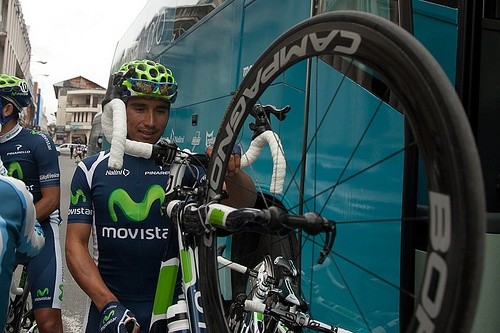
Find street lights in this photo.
[15,60,47,78]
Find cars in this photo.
[54,143,86,156]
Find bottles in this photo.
[166,299,190,333]
[165,200,179,217]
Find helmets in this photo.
[113,59,177,103]
[0,73,32,113]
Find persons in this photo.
[63,61,256,333]
[0,74,64,333]
[70,145,87,159]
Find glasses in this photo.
[125,77,178,96]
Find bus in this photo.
[104,0,500,332]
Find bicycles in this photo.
[99,9,486,333]
